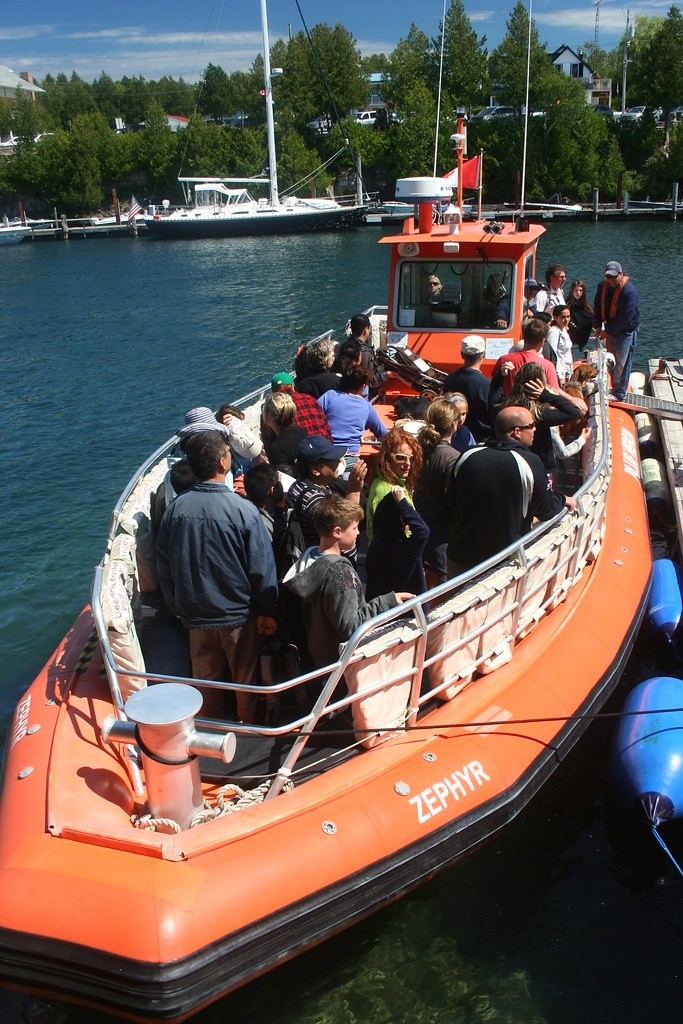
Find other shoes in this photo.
[608,393,621,401]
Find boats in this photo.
[0,110,661,1024]
[502,203,584,212]
[382,201,472,217]
[0,211,152,244]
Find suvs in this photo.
[588,105,613,119]
[470,105,521,122]
[348,111,379,124]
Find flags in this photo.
[127,196,144,227]
[443,154,481,189]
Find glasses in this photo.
[391,453,415,464]
[606,272,620,280]
[554,274,566,279]
[508,420,536,431]
[487,279,502,286]
[260,404,268,413]
[428,282,439,286]
[217,444,232,465]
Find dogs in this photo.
[561,364,600,438]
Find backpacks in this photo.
[275,485,328,569]
[253,641,328,733]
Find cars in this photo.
[116,124,145,135]
[522,106,549,118]
[0,132,53,146]
[615,106,683,121]
[202,116,266,127]
[307,114,331,132]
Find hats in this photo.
[461,336,485,355]
[272,371,298,394]
[176,406,229,439]
[604,261,622,276]
[525,278,540,292]
[297,434,348,467]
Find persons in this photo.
[592,262,641,400]
[152,263,592,730]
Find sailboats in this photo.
[144,1,381,237]
